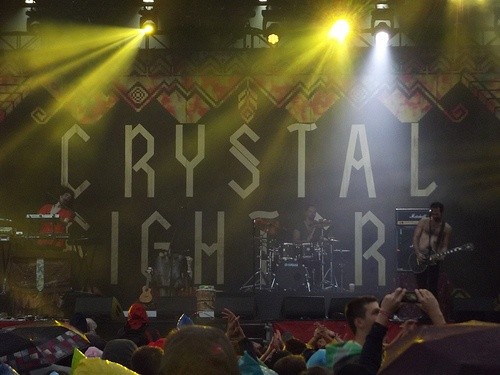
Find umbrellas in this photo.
[0,314,93,373]
[375,319,500,375]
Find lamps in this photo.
[25,6,43,34]
[371,2,394,42]
[261,1,284,45]
[138,2,160,35]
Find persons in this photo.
[0,284,500,375]
[294,206,329,243]
[33,184,77,257]
[410,198,454,294]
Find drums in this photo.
[297,242,315,259]
[277,241,296,261]
[274,260,312,293]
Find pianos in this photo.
[9,212,102,295]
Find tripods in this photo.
[323,243,337,289]
[241,245,270,293]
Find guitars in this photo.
[408,242,475,273]
[138,268,153,303]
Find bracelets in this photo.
[378,307,394,320]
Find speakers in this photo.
[74,296,124,319]
[216,297,260,319]
[279,295,376,319]
[396,226,431,292]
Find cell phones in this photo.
[401,292,417,303]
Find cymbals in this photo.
[309,221,331,226]
[261,222,287,235]
